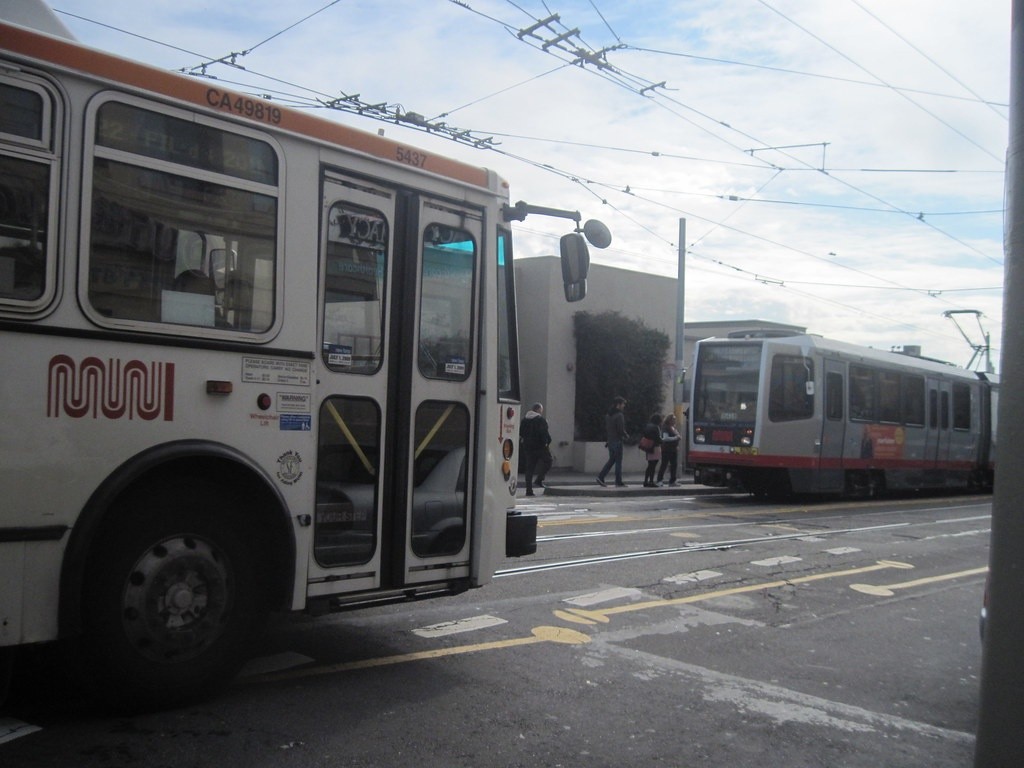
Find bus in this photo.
[0,0,611,725]
[678,308,1000,508]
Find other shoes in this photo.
[616,483,625,487]
[644,482,648,487]
[648,483,659,487]
[596,476,607,487]
[657,482,663,486]
[534,479,546,488]
[526,490,534,496]
[669,482,681,486]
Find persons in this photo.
[178,269,234,331]
[519,403,553,495]
[657,414,681,486]
[597,396,629,487]
[644,413,660,487]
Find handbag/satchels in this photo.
[639,436,656,453]
